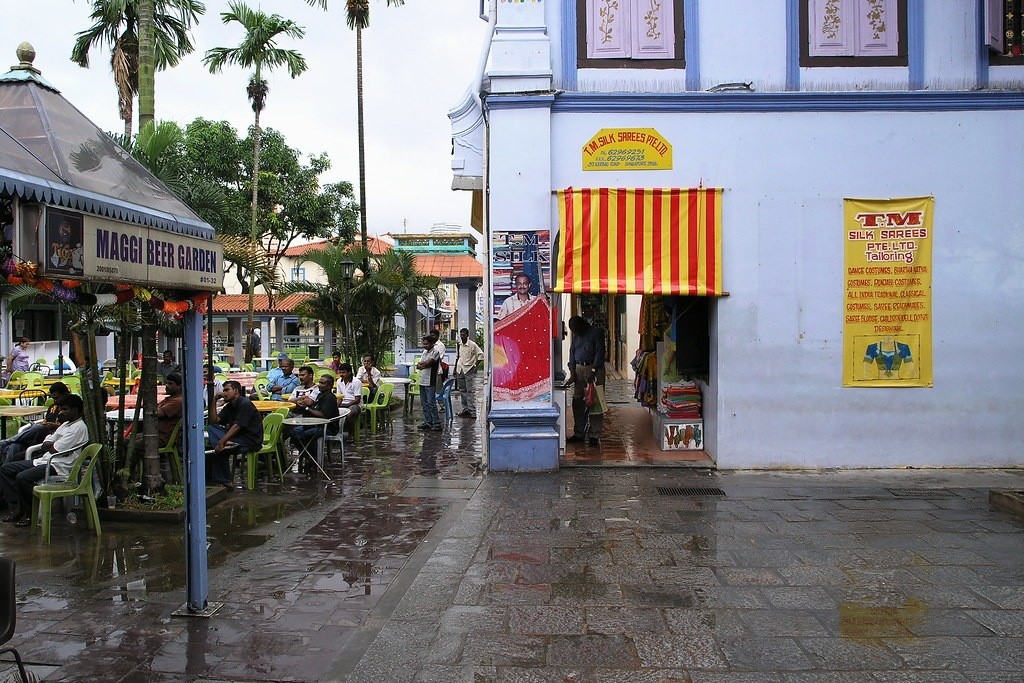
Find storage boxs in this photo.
[652,410,705,451]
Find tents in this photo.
[0,42,225,618]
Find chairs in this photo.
[0,347,455,545]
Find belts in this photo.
[577,361,593,365]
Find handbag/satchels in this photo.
[441,362,449,378]
[584,381,607,415]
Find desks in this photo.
[0,406,49,440]
[381,378,411,427]
[105,395,170,437]
[104,379,137,395]
[225,373,257,378]
[0,389,50,405]
[251,400,296,474]
[104,408,143,481]
[8,380,60,402]
[229,378,256,394]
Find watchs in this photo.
[591,368,597,372]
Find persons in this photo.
[111,349,263,486]
[661,301,676,383]
[415,328,445,431]
[248,351,381,469]
[564,316,606,446]
[3,337,30,388]
[215,326,261,365]
[498,272,550,321]
[0,382,108,527]
[454,328,483,418]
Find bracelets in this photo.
[305,407,308,411]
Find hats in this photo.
[278,353,287,359]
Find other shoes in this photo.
[1,510,22,521]
[455,412,470,417]
[216,482,234,490]
[431,427,443,431]
[589,437,599,446]
[218,443,241,456]
[16,513,31,527]
[567,434,585,440]
[347,433,354,442]
[417,423,433,429]
[471,413,477,417]
